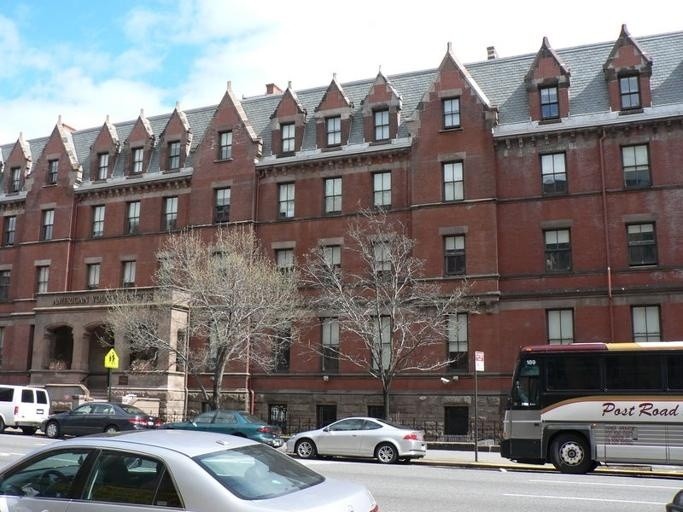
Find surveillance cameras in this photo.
[440,378,450,385]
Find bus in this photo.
[499,340,683,473]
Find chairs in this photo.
[244,465,267,480]
[98,455,128,475]
